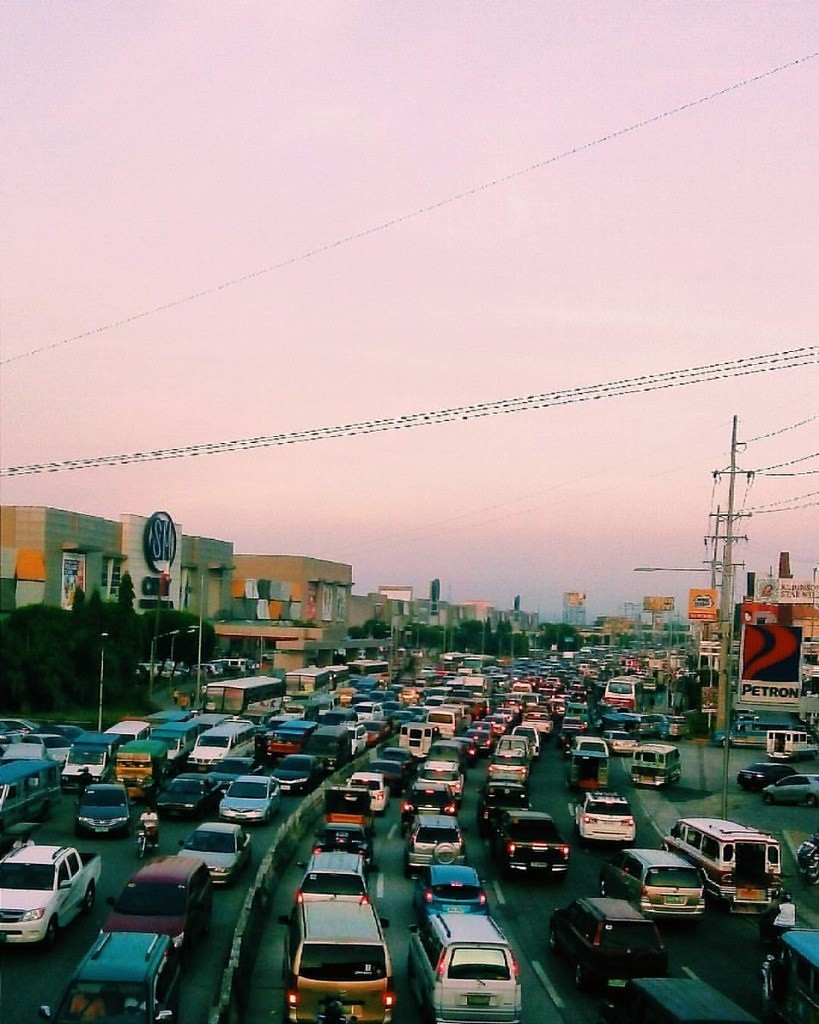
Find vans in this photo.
[277,899,396,1024]
[407,913,522,1023]
[632,743,682,788]
[105,720,152,752]
[0,759,61,834]
[602,967,762,1024]
[61,731,122,793]
[660,817,782,915]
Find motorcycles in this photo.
[137,821,155,858]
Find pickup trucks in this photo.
[496,810,571,877]
[1,844,100,953]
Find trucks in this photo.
[712,721,794,750]
[766,729,818,762]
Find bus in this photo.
[769,929,819,1024]
[115,739,168,803]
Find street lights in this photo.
[150,631,177,699]
[97,632,108,731]
[634,566,734,824]
[169,629,197,693]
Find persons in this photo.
[76,767,93,808]
[172,687,179,703]
[201,684,208,695]
[139,804,161,847]
[180,693,189,711]
[12,830,34,850]
[190,689,196,706]
[761,929,793,1017]
[759,888,797,928]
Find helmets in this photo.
[783,892,792,902]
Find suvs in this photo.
[403,814,468,877]
[477,782,532,836]
[399,781,461,825]
[412,864,492,925]
[549,897,671,990]
[311,823,377,871]
[599,848,707,928]
[293,852,372,904]
[37,931,182,1024]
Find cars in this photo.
[176,822,251,887]
[736,762,799,792]
[568,790,636,847]
[218,776,283,826]
[137,643,693,819]
[324,786,376,833]
[0,718,87,766]
[100,855,213,968]
[762,774,819,808]
[156,773,223,821]
[73,783,136,837]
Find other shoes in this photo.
[154,844,158,848]
